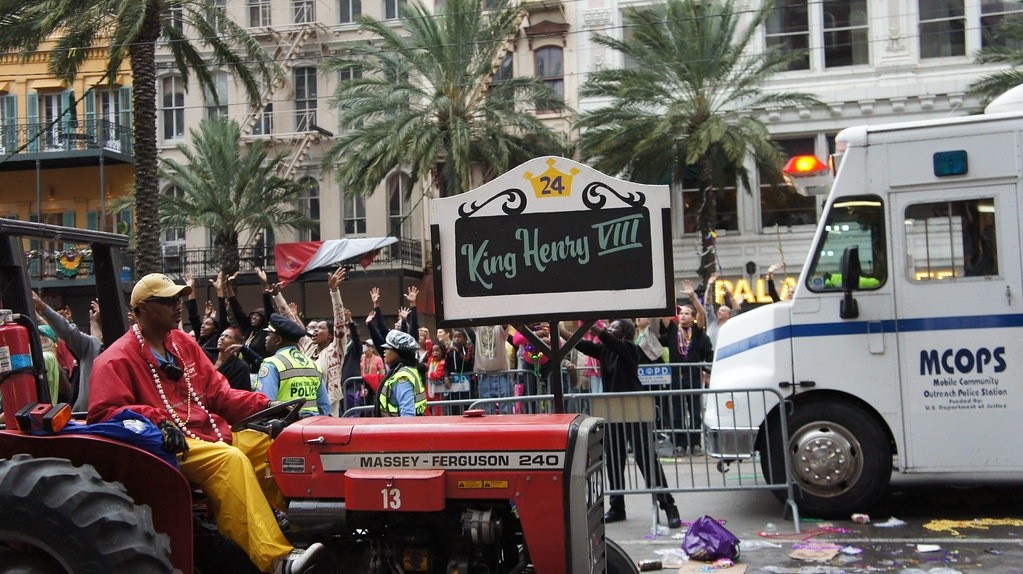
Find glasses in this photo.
[143,295,184,306]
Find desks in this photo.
[62,133,92,139]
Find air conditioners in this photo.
[162,245,179,258]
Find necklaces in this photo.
[132,323,223,441]
[487,326,494,360]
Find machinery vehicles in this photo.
[0,217,639,574]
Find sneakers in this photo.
[274,542,325,574]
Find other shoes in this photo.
[605,508,626,523]
[665,505,681,528]
[693,445,703,456]
[676,446,689,457]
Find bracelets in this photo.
[766,276,774,280]
[688,294,694,299]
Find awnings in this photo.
[87,75,123,87]
[31,79,66,90]
[0,82,9,93]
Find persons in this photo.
[340,309,362,416]
[559,319,681,529]
[635,273,740,457]
[360,339,385,417]
[766,265,795,302]
[181,267,346,416]
[375,329,426,416]
[472,324,510,416]
[366,285,477,416]
[505,320,606,414]
[32,291,103,414]
[86,274,324,574]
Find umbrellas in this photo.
[68,91,78,140]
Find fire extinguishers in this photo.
[0,309,47,430]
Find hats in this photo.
[38,324,57,342]
[129,272,193,311]
[249,307,266,315]
[380,329,421,353]
[362,338,375,348]
[262,313,305,343]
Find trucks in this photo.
[701,83,1022,519]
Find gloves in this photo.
[157,419,190,462]
[266,399,302,423]
